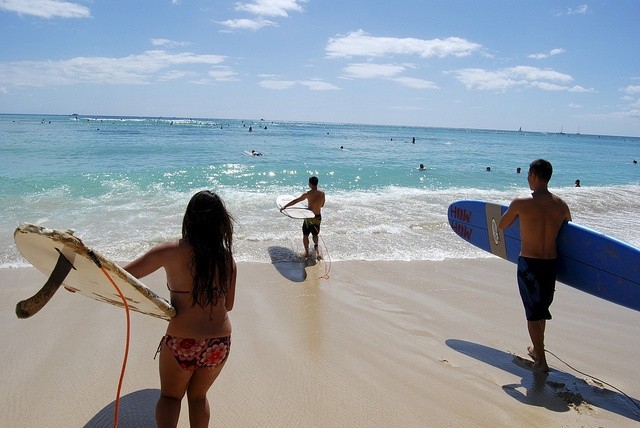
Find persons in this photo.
[419,164,426,170]
[486,166,490,171]
[412,136,416,143]
[252,150,262,156]
[499,159,572,372]
[574,179,580,187]
[340,145,343,149]
[517,168,521,173]
[63,190,237,427]
[279,177,325,256]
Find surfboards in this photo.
[276,194,315,219]
[15,222,178,321]
[447,200,640,312]
